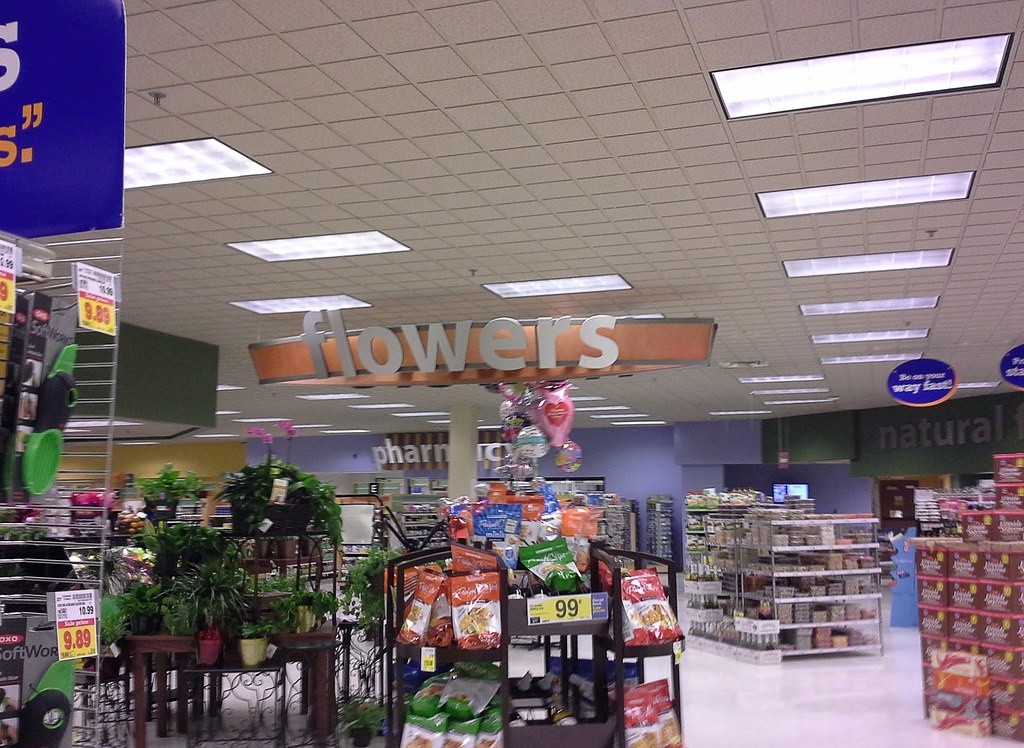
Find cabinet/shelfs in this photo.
[384,540,682,748]
[681,491,884,666]
[338,544,374,583]
[401,501,449,552]
[289,536,339,583]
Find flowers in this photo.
[212,418,296,509]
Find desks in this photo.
[86,555,341,748]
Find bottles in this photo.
[685,563,722,582]
[687,594,718,610]
[739,633,778,651]
[690,621,738,647]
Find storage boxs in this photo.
[914,452,1024,742]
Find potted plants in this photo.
[170,558,252,669]
[236,621,274,667]
[339,697,387,748]
[68,449,405,636]
[82,608,127,682]
